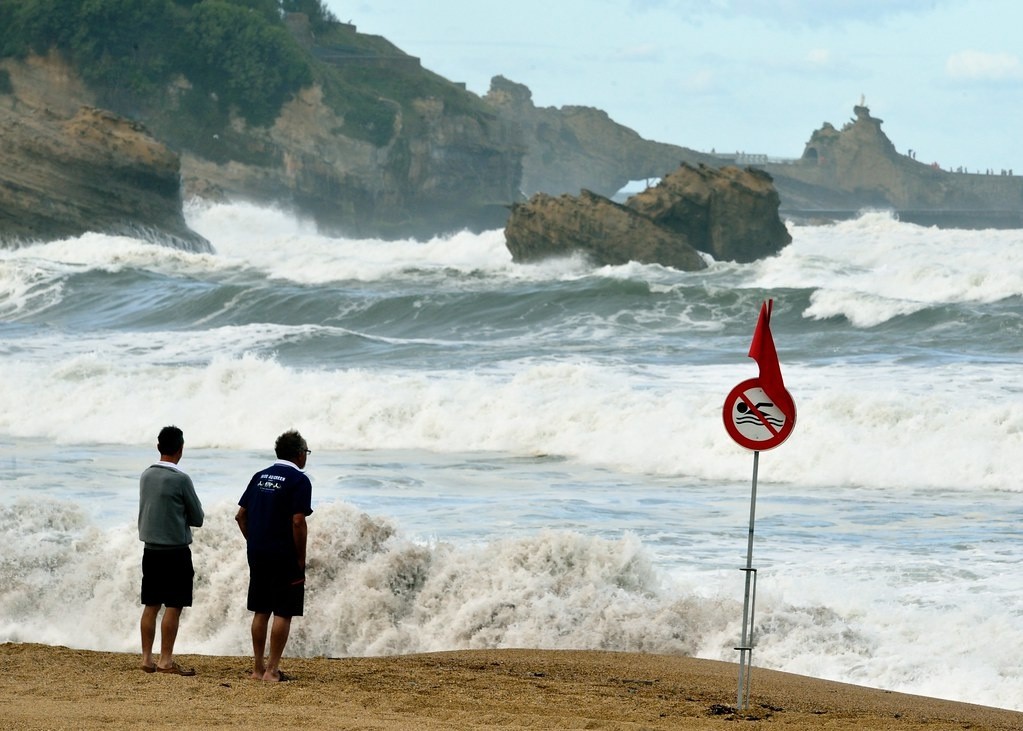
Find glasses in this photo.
[303,449,311,455]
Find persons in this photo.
[139,426,205,676]
[235,431,314,682]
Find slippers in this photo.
[277,670,292,681]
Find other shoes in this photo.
[157,660,195,676]
[141,662,158,673]
